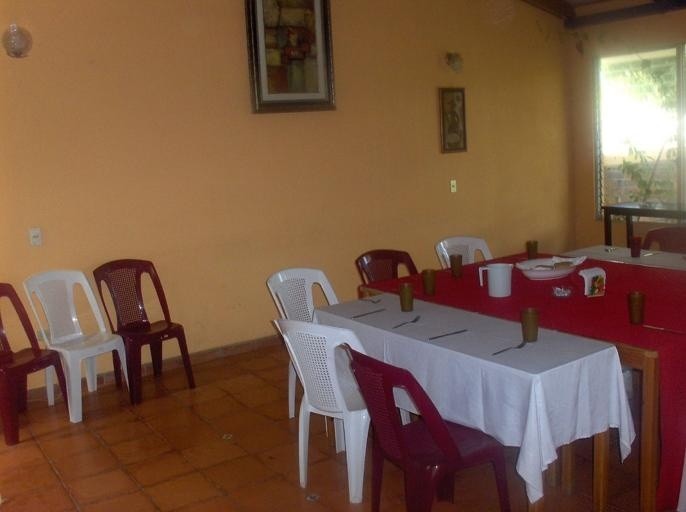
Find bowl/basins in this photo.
[515,255,587,281]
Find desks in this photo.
[600,200,685,250]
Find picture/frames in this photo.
[243,0,336,113]
[438,85,466,153]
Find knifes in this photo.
[643,324,686,336]
[642,251,661,257]
[426,329,469,340]
[350,308,388,319]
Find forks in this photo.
[490,342,526,356]
[390,314,420,329]
[357,297,381,304]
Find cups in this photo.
[450,254,462,278]
[627,291,645,324]
[521,307,539,343]
[630,235,641,258]
[525,240,538,259]
[397,283,414,312]
[550,279,573,297]
[603,240,616,257]
[420,269,437,295]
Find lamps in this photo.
[1,24,31,58]
[442,48,464,74]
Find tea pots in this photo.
[476,263,515,298]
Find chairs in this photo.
[0,283,68,449]
[20,270,132,427]
[94,259,193,404]
[643,226,685,251]
[267,267,340,419]
[273,318,410,504]
[340,342,511,512]
[355,249,418,283]
[436,236,493,269]
